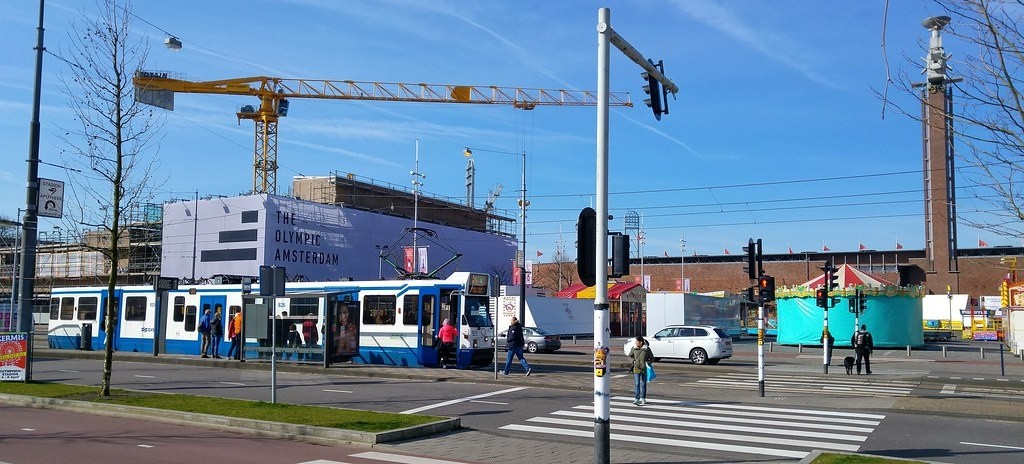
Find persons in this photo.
[498,316,531,377]
[199,309,247,361]
[259,311,319,362]
[821,324,873,375]
[375,308,460,369]
[628,336,655,405]
[332,303,357,356]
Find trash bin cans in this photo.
[81,323,92,351]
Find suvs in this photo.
[623,325,733,365]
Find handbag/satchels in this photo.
[198,320,204,332]
[646,362,657,382]
[629,366,633,373]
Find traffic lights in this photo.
[832,297,841,307]
[742,238,755,279]
[830,268,839,290]
[574,207,596,287]
[859,290,867,314]
[741,287,754,302]
[849,299,856,313]
[638,59,661,122]
[816,290,826,306]
[758,276,775,302]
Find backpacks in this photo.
[856,331,869,348]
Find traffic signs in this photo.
[37,178,65,219]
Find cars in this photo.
[494,326,561,353]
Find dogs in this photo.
[844,357,855,375]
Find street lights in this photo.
[462,146,527,327]
[15,0,182,383]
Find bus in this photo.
[47,228,495,370]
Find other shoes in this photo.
[443,365,447,369]
[633,399,640,404]
[642,399,646,404]
[213,356,215,358]
[215,355,221,358]
[857,371,860,374]
[525,367,532,376]
[202,354,210,358]
[226,357,230,360]
[867,371,872,375]
[499,370,508,377]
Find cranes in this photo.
[131,69,633,194]
